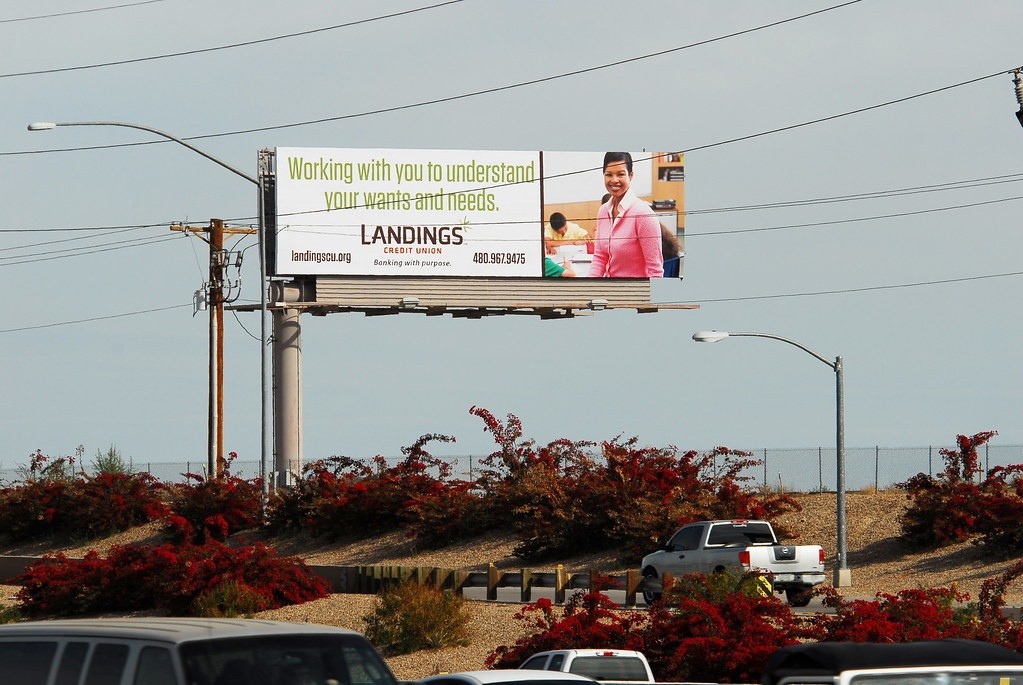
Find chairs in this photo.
[662,255,681,278]
[278,650,325,685]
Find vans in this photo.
[516,648,655,685]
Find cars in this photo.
[420,669,605,685]
[751,638,1023,685]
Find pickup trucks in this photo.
[641,518,826,608]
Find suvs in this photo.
[0,615,402,684]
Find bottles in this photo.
[585,241,595,254]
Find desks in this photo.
[652,208,678,238]
[545,246,592,277]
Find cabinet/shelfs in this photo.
[652,152,684,229]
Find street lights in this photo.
[27,122,272,525]
[691,329,851,588]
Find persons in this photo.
[602,194,680,278]
[588,152,664,278]
[544,212,592,277]
